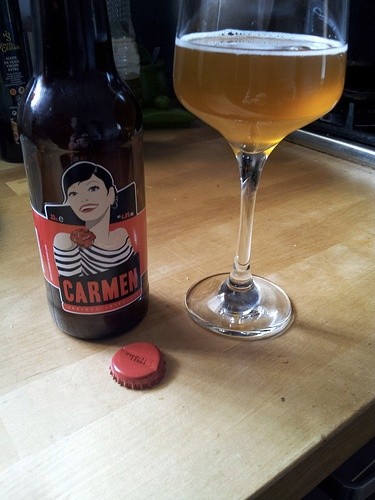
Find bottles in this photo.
[19,0,149,340]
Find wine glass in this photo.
[174,0,351,340]
